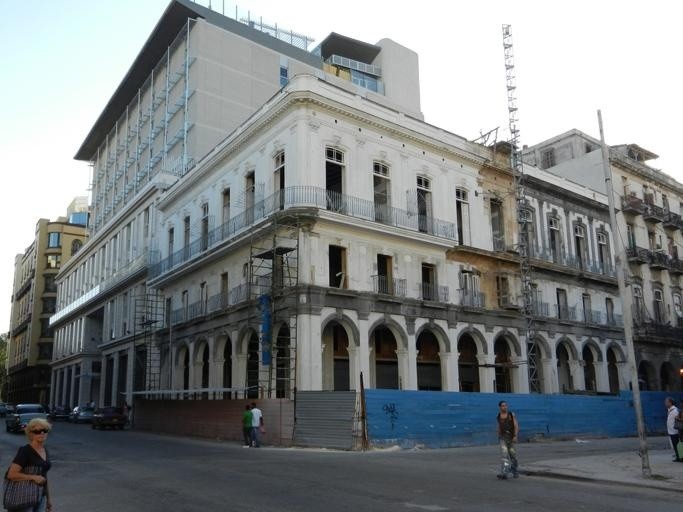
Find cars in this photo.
[0,402,127,433]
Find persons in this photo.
[4,417,52,512]
[241,405,254,449]
[250,401,264,448]
[496,400,521,479]
[88,400,95,410]
[123,401,130,425]
[665,395,683,463]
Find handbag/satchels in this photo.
[3,464,44,510]
[676,441,683,459]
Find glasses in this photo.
[29,427,48,434]
